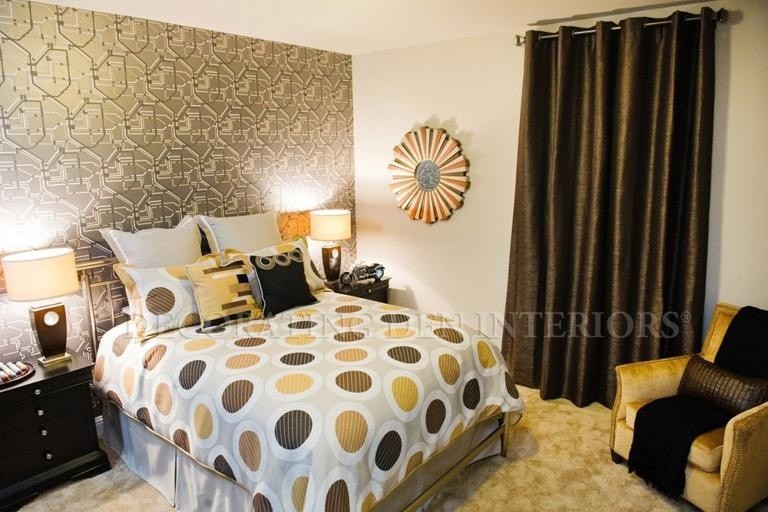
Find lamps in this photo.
[309,209,352,285]
[1,247,80,368]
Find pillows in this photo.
[223,234,334,294]
[183,256,262,331]
[250,247,319,319]
[194,211,283,255]
[112,254,223,342]
[99,214,202,265]
[247,272,265,307]
[678,353,768,413]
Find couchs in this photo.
[610,304,768,512]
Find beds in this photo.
[76,256,526,512]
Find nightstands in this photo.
[334,277,390,303]
[0,347,111,512]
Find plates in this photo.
[0,361,35,386]
